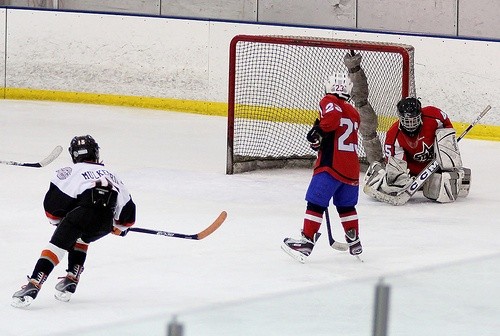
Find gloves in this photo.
[112,226,130,236]
[307,127,324,151]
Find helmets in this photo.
[68,135,100,164]
[325,73,354,100]
[396,98,421,137]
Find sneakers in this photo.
[8,282,41,308]
[53,274,79,302]
[345,228,364,263]
[281,231,322,263]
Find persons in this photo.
[283,72,365,257]
[7,135,138,302]
[384,98,455,191]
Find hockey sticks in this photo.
[129,211,229,241]
[363,104,492,207]
[312,139,349,252]
[0,146,63,167]
